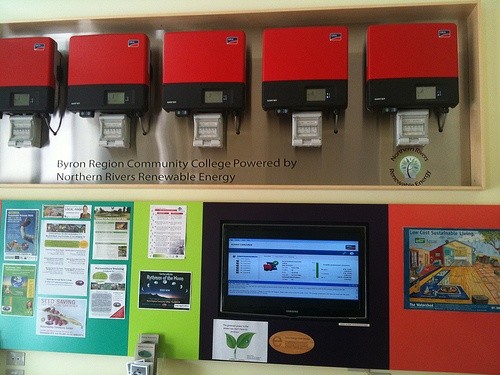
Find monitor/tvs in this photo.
[219,223,370,322]
